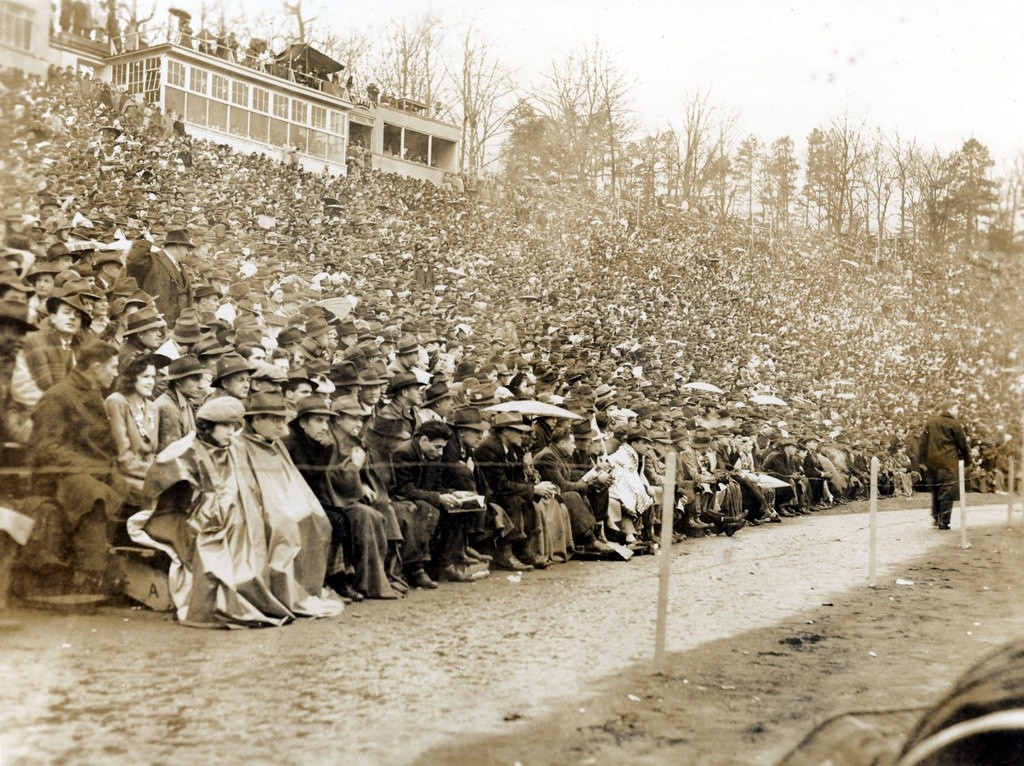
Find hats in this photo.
[0,64,814,443]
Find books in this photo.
[447,495,487,513]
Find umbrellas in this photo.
[683,383,722,393]
[315,297,352,320]
[749,395,788,406]
[478,400,585,447]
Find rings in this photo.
[450,504,454,508]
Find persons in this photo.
[917,399,977,529]
[0,0,1024,632]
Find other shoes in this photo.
[185,484,946,632]
